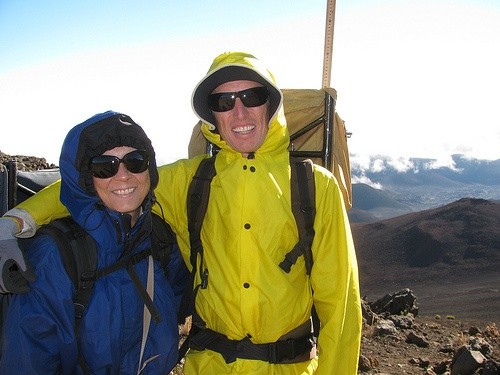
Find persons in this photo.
[0,110,194,375]
[0,51,362,375]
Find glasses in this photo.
[87,149,150,179]
[204,86,270,113]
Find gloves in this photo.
[1,218,39,292]
